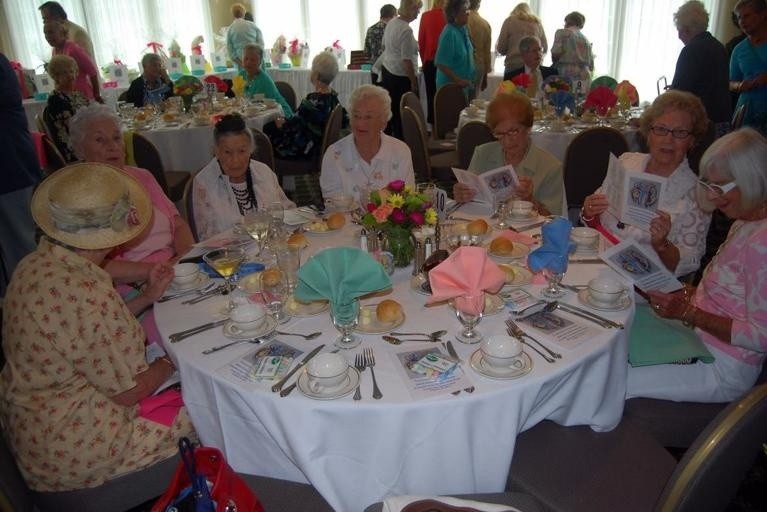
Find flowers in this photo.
[351,179,443,263]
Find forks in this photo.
[353,353,367,401]
[503,319,563,364]
[364,346,384,400]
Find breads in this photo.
[262,269,281,286]
[134,112,149,122]
[491,237,513,255]
[161,114,176,121]
[144,105,154,113]
[498,266,515,283]
[327,214,345,230]
[288,234,307,251]
[376,300,403,321]
[467,220,488,235]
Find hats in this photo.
[31,162,153,250]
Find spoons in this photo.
[379,335,443,345]
[390,329,448,338]
[275,330,323,341]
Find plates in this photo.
[295,364,361,400]
[319,201,361,212]
[451,223,494,236]
[166,270,211,293]
[502,212,538,221]
[278,295,329,318]
[350,302,406,335]
[444,293,506,317]
[469,348,534,380]
[572,248,601,257]
[492,263,534,285]
[221,313,280,340]
[487,240,530,259]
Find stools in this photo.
[234,468,333,511]
[364,493,545,512]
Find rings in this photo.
[653,304,662,311]
[590,206,591,208]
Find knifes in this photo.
[576,288,631,311]
[270,344,325,394]
[554,301,625,330]
[441,340,475,395]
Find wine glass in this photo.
[203,201,302,325]
[539,263,570,298]
[454,297,484,344]
[327,298,362,349]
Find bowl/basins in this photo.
[445,233,481,252]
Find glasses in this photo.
[650,127,691,139]
[493,129,518,139]
[698,179,736,195]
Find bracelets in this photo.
[156,355,175,372]
[581,207,594,221]
[681,303,691,322]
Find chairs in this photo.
[1,434,186,512]
[398,71,651,206]
[623,356,767,449]
[500,382,765,510]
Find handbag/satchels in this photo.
[151,436,262,512]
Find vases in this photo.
[377,231,420,267]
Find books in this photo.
[218,339,305,392]
[598,232,686,295]
[390,342,471,404]
[515,305,603,350]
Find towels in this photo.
[627,303,717,369]
[527,216,582,277]
[293,244,395,324]
[423,244,509,315]
[170,74,246,101]
[196,260,265,280]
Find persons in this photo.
[2,2,337,192]
[621,124,767,407]
[671,0,766,170]
[192,112,296,243]
[365,0,593,179]
[0,159,202,512]
[321,85,414,209]
[576,79,713,277]
[454,91,563,217]
[68,103,193,350]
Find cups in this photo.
[508,201,533,216]
[172,263,201,284]
[321,193,355,209]
[228,303,266,335]
[588,277,631,302]
[567,227,600,248]
[464,95,632,134]
[479,334,526,370]
[115,92,278,130]
[306,352,350,393]
